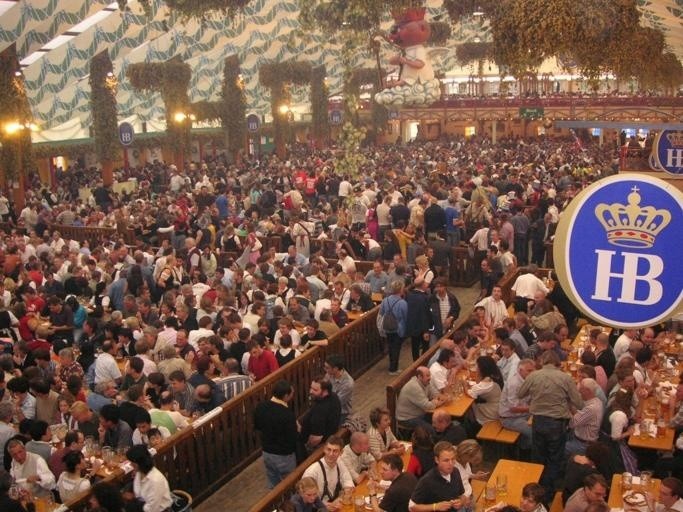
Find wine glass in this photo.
[584,324,592,344]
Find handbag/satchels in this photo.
[156,268,174,289]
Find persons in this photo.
[2,131,683,511]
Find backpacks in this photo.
[383,297,403,330]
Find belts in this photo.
[573,434,592,445]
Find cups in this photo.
[350,303,358,316]
[483,474,507,506]
[9,483,56,512]
[84,434,118,471]
[619,471,652,494]
[634,332,682,440]
[447,348,494,400]
[340,486,366,512]
[566,343,583,377]
[327,282,334,290]
